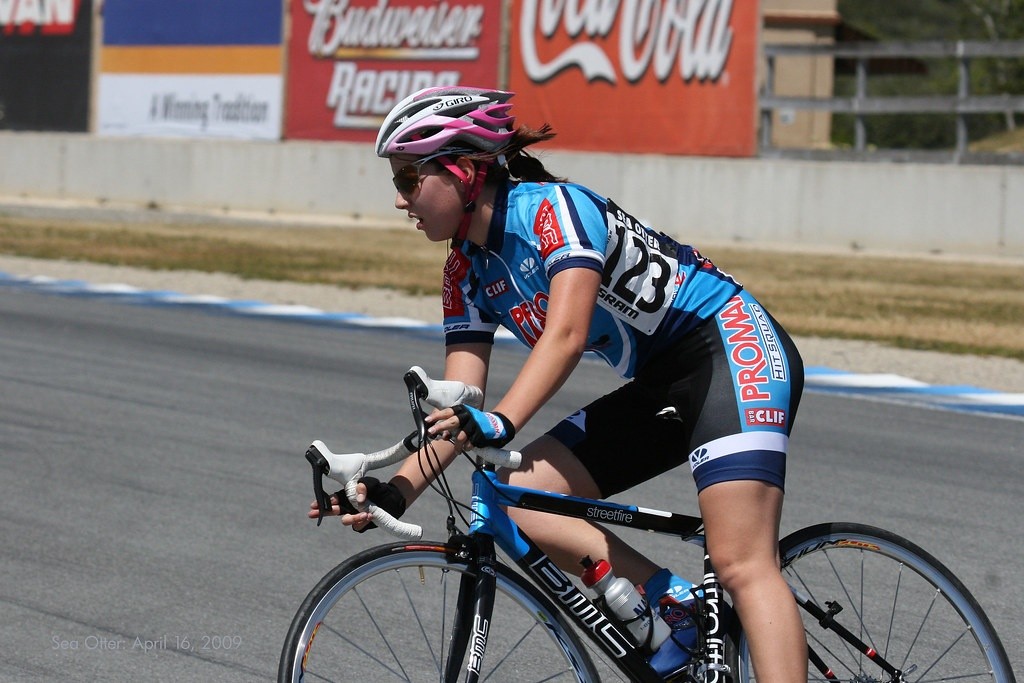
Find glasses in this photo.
[392,150,472,194]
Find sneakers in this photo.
[642,567,712,679]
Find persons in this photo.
[310,86,809,683]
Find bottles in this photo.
[579,555,672,652]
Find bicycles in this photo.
[276,363,1016,683]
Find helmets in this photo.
[374,86,521,158]
